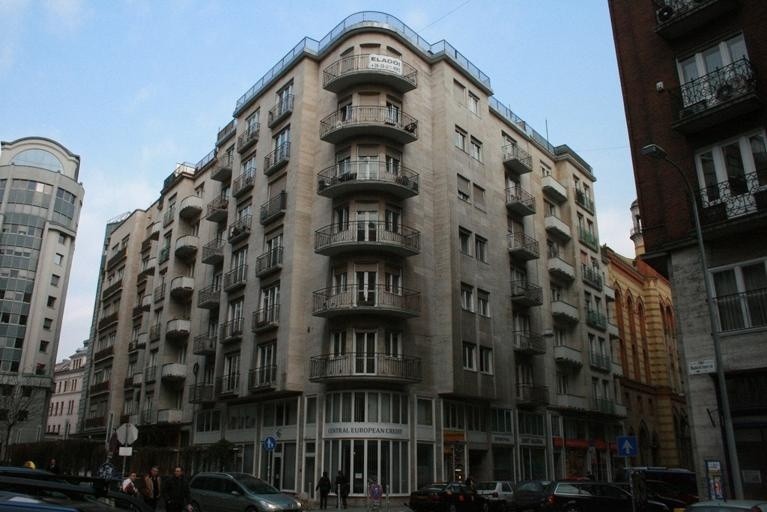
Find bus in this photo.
[615,466,698,512]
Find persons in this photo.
[47,459,60,474]
[163,466,193,512]
[315,471,332,510]
[334,471,349,509]
[120,471,138,496]
[142,464,162,512]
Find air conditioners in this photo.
[654,3,678,25]
[678,73,748,122]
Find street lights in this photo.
[641,143,745,499]
